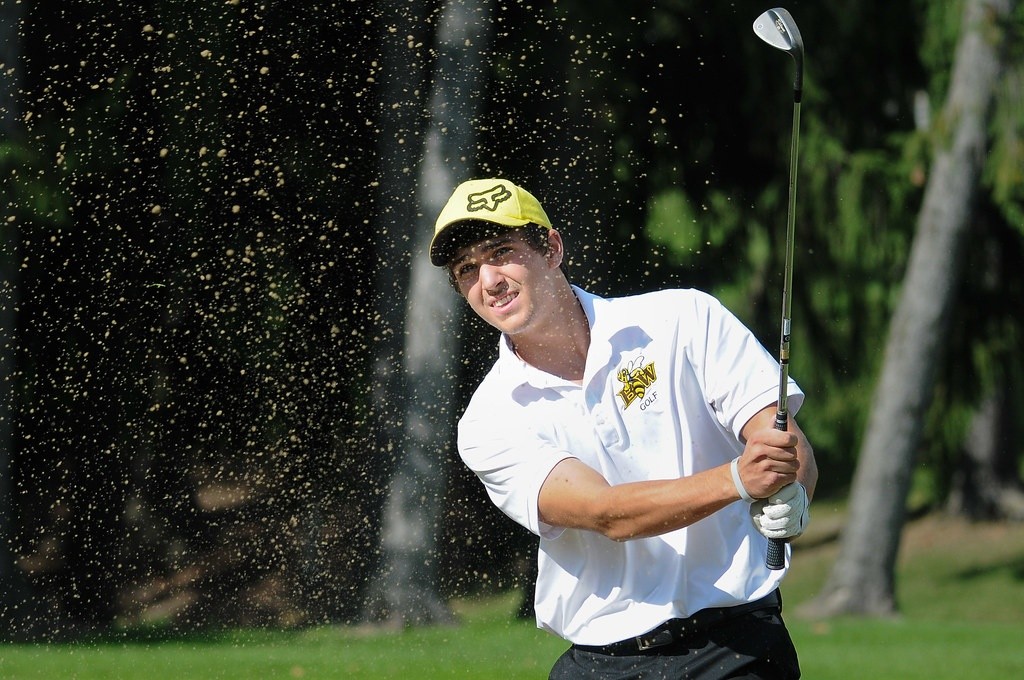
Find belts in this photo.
[594,589,782,654]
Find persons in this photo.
[429,179,818,680]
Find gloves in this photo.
[749,479,812,540]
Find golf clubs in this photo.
[751,7,805,572]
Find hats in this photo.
[430,178,554,268]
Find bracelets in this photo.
[730,456,758,503]
